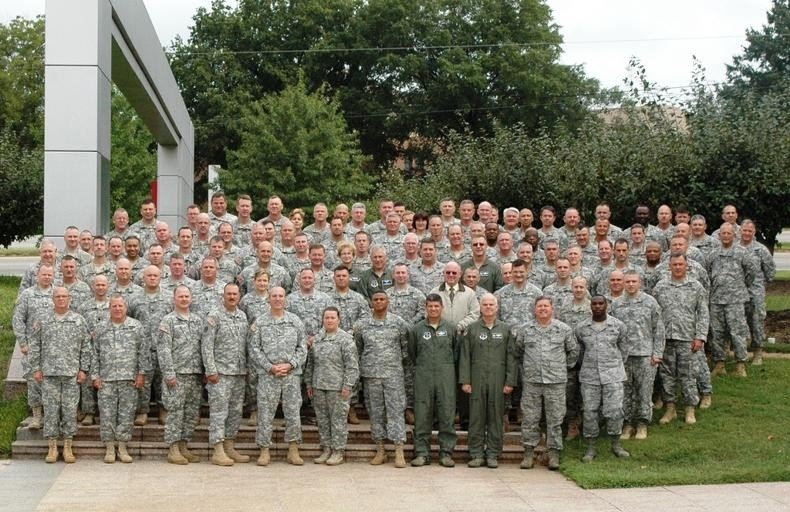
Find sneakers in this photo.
[411,456,431,466]
[135,413,148,425]
[81,413,94,425]
[620,424,634,439]
[212,439,250,465]
[247,411,257,426]
[565,425,579,440]
[736,361,747,377]
[349,408,359,423]
[711,362,726,375]
[158,407,167,425]
[636,421,647,439]
[468,459,484,467]
[487,457,497,467]
[439,454,455,466]
[314,447,343,464]
[653,395,711,424]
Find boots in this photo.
[370,445,388,464]
[257,447,270,465]
[286,441,303,465]
[395,444,406,468]
[752,348,763,365]
[548,449,560,469]
[29,406,42,429]
[63,439,76,462]
[520,447,533,468]
[168,439,200,464]
[104,441,116,463]
[582,437,598,461]
[117,441,133,462]
[612,435,630,457]
[45,437,58,462]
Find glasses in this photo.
[474,242,484,245]
[446,271,457,275]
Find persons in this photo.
[12,192,777,470]
